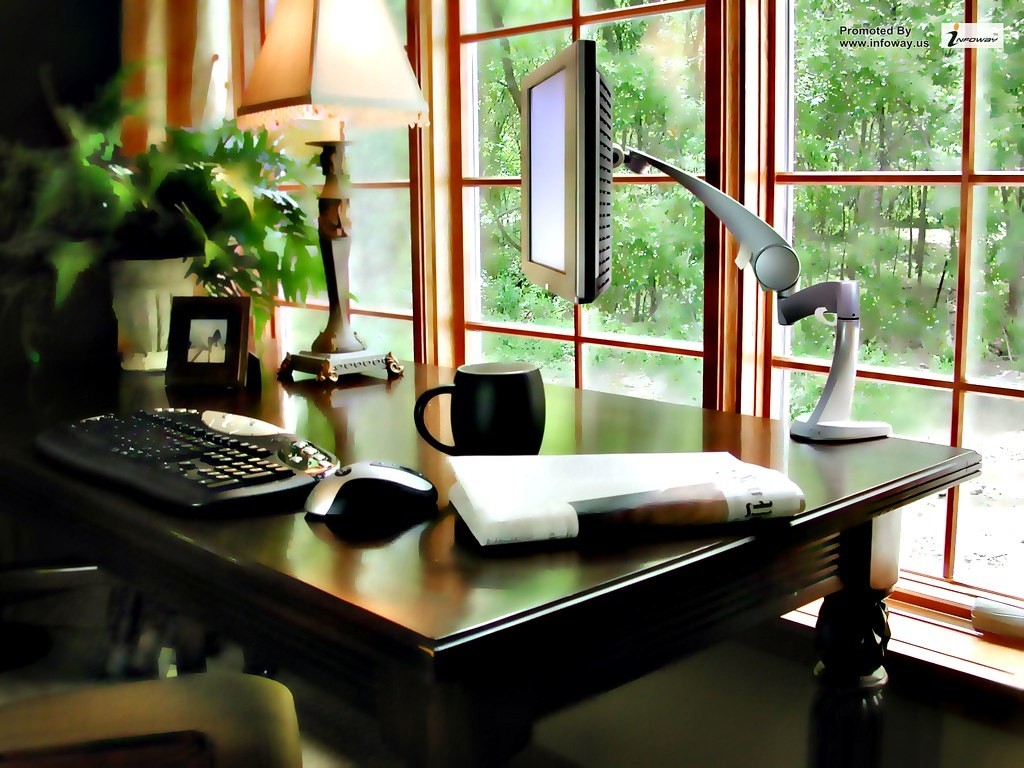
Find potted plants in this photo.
[0,55,327,377]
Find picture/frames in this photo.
[161,295,251,386]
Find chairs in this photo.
[1,561,410,768]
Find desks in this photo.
[1,338,979,768]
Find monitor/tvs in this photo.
[521,39,614,302]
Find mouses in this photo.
[305,460,440,519]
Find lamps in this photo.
[234,0,431,385]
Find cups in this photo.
[414,362,545,455]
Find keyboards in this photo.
[35,408,341,519]
